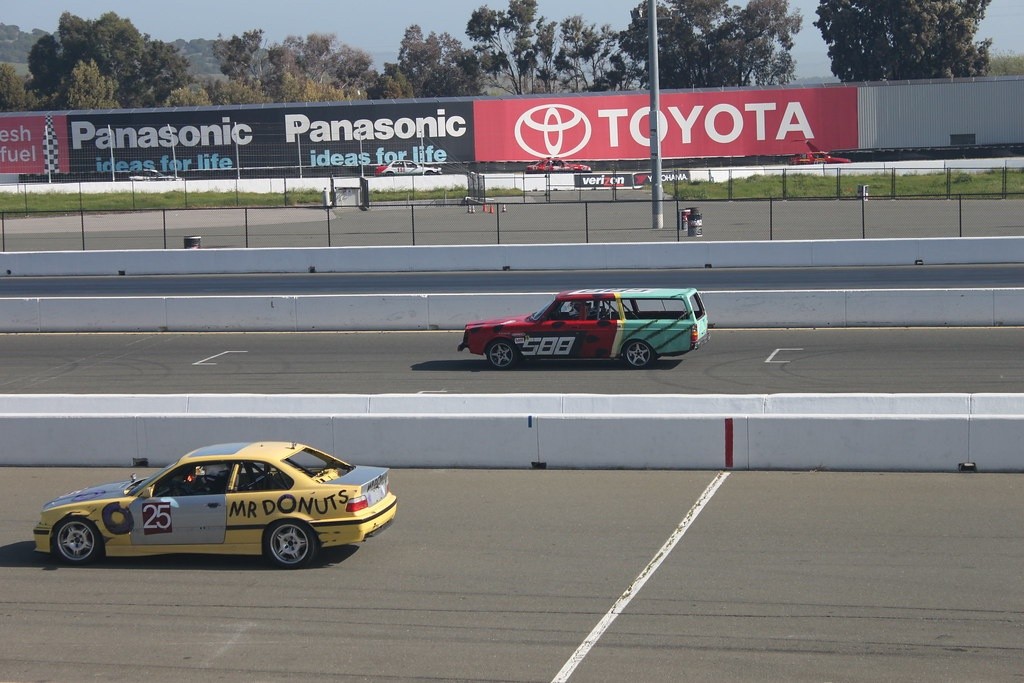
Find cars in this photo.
[129,169,185,181]
[526,157,592,174]
[457,287,711,370]
[791,152,850,165]
[33,441,397,568]
[375,160,442,176]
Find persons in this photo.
[200,464,242,492]
[568,300,589,319]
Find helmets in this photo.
[568,301,584,316]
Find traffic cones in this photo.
[472,205,475,213]
[468,205,471,213]
[502,204,506,212]
